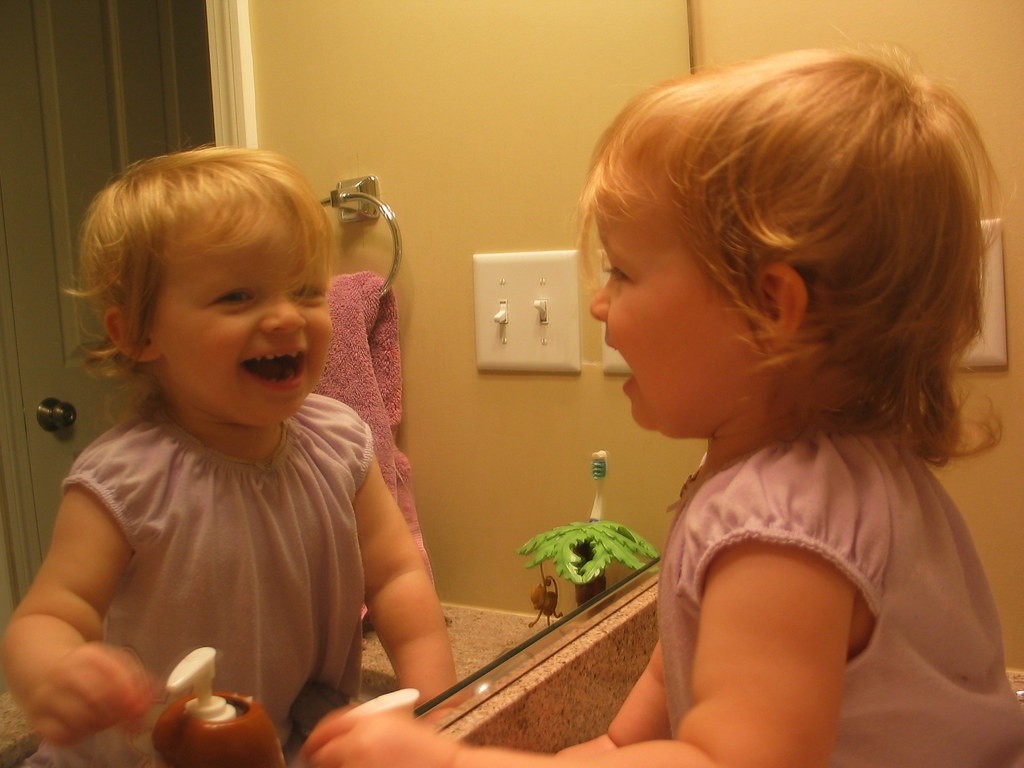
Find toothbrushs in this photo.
[587,449,609,525]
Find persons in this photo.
[6,145,457,768]
[293,40,1024,768]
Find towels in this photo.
[305,272,424,623]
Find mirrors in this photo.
[0,0,708,768]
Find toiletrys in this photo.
[149,645,285,768]
